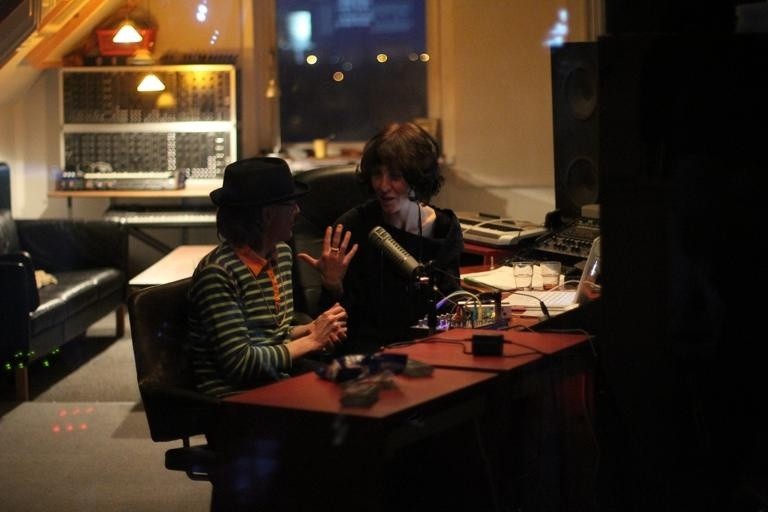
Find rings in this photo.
[330,247,340,253]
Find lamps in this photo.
[112,1,142,44]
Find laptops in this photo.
[501,237,602,310]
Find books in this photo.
[458,264,579,320]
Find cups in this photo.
[539,261,562,290]
[511,260,533,292]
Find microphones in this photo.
[368,226,425,281]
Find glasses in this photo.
[269,202,296,216]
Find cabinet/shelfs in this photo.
[58,63,238,188]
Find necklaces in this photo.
[229,242,287,327]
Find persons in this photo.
[164,153,349,512]
[294,118,465,362]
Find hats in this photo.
[209,157,310,208]
